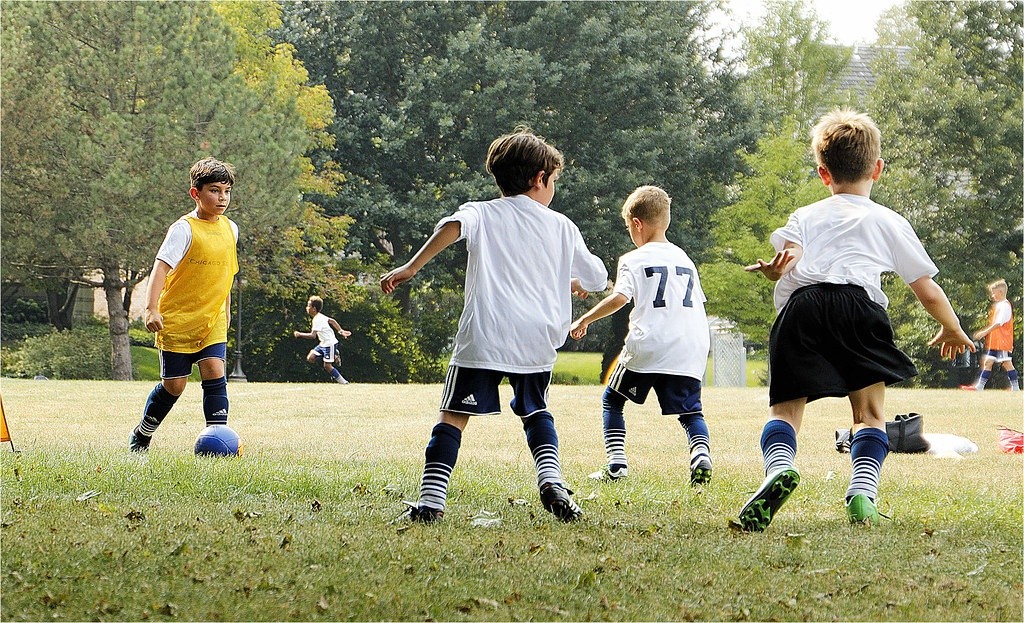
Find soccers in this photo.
[194,424,244,459]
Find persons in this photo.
[293,294,351,385]
[961,279,1020,393]
[127,156,239,459]
[567,184,712,489]
[380,126,608,534]
[737,106,976,537]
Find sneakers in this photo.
[739,466,800,533]
[392,501,444,525]
[130,425,149,457]
[334,349,341,367]
[845,494,892,528]
[540,481,583,523]
[603,463,628,484]
[961,384,977,391]
[690,455,712,489]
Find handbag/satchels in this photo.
[836,430,851,451]
[886,413,931,452]
